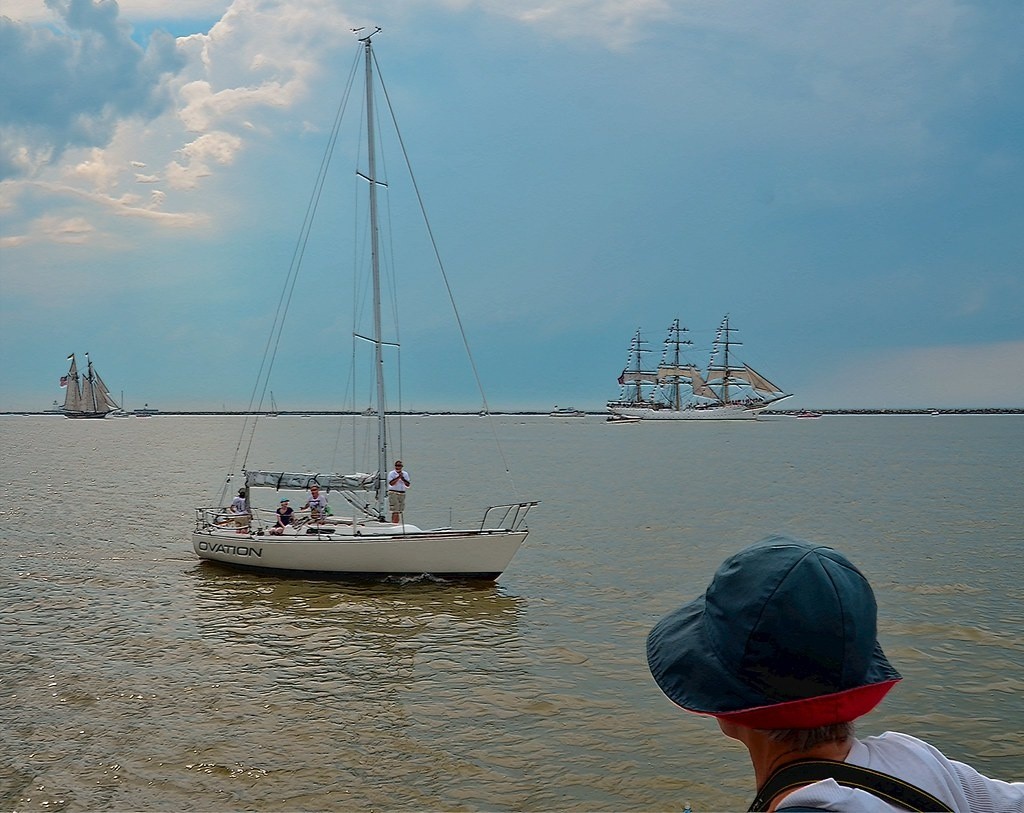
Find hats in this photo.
[238,488,245,493]
[280,497,290,503]
[647,536,904,728]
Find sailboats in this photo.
[189,25,543,585]
[57,352,160,420]
[604,311,795,421]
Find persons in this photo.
[230,488,247,533]
[300,486,328,524]
[647,534,1024,813]
[388,461,410,523]
[269,497,295,536]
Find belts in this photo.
[390,490,405,493]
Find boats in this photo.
[797,410,823,418]
[549,409,587,418]
[605,413,643,424]
